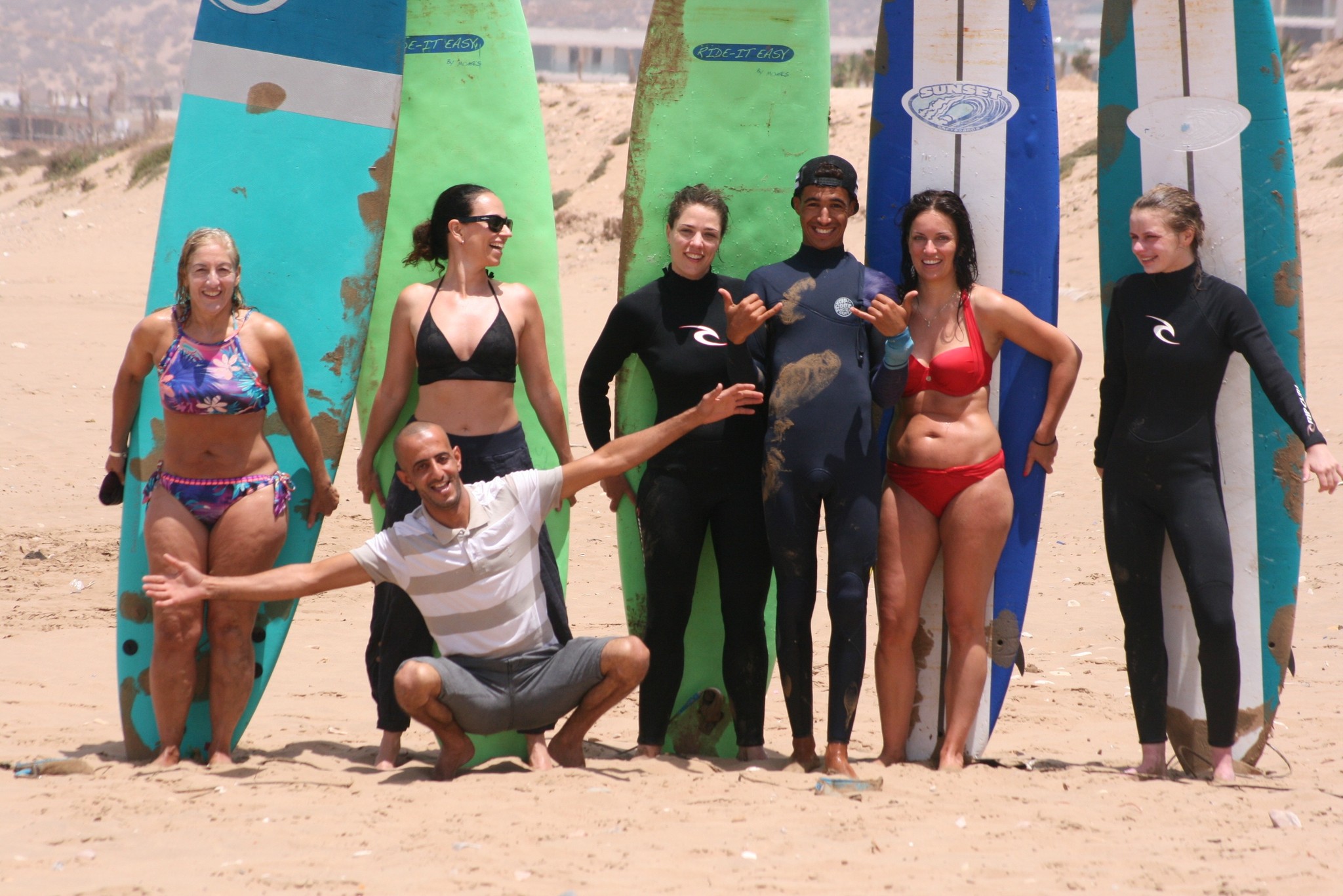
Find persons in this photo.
[356,184,578,775]
[1093,186,1341,786]
[105,227,339,776]
[141,383,765,781]
[579,184,774,761]
[874,187,1082,772]
[719,156,918,778]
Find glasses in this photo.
[460,215,513,232]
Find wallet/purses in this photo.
[99,471,123,506]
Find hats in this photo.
[791,155,860,216]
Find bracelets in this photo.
[108,445,127,458]
[1032,436,1056,446]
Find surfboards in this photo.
[864,1,1061,767]
[114,0,570,765]
[1094,2,1309,779]
[612,0,831,766]
